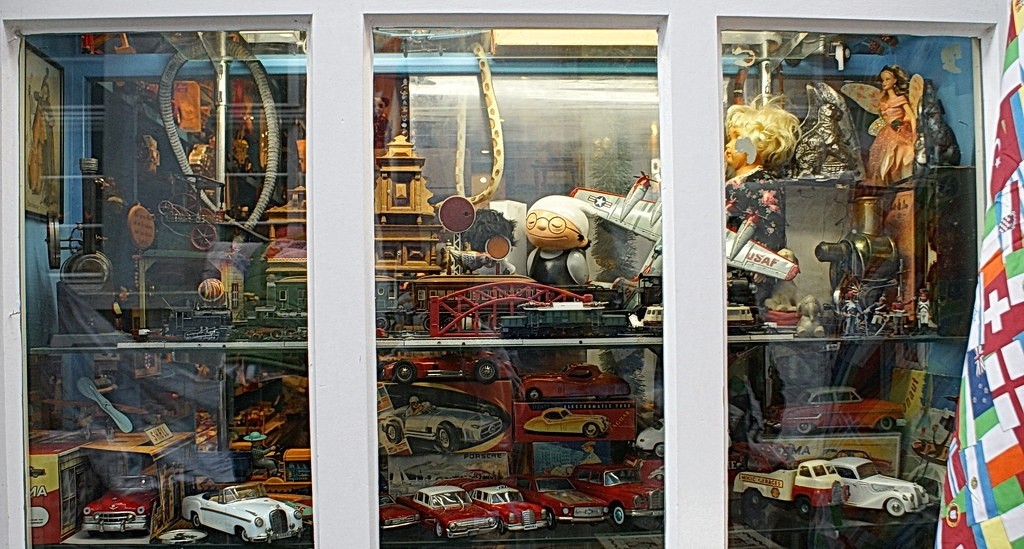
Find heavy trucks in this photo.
[732,459,846,520]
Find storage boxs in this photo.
[61,432,196,545]
[885,165,977,336]
[761,430,902,478]
[388,450,509,503]
[512,401,637,441]
[377,381,516,453]
[519,441,612,480]
[29,429,106,545]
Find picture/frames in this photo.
[24,40,64,225]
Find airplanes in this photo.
[41,375,149,440]
[570,171,800,310]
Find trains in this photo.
[161,273,540,341]
[495,301,756,339]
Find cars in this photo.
[379,492,419,531]
[763,385,906,433]
[181,482,303,543]
[501,472,609,529]
[571,462,664,525]
[80,474,158,535]
[518,361,632,401]
[635,419,733,456]
[828,457,933,518]
[466,484,548,534]
[382,350,517,383]
[396,485,499,539]
